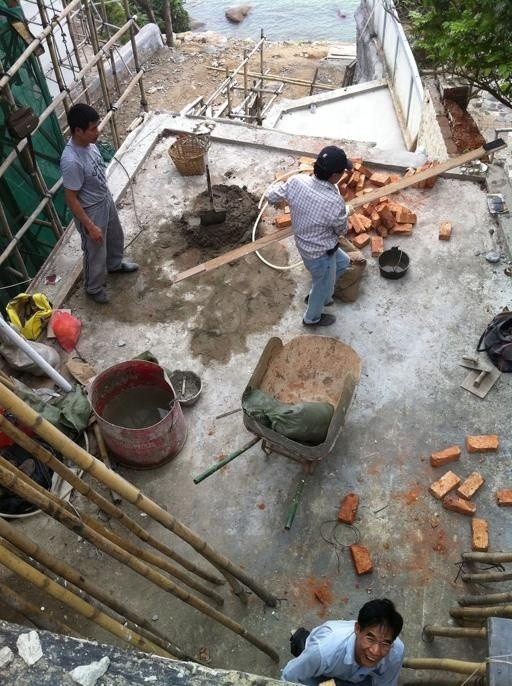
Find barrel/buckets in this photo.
[89,359,189,470]
[378,246,410,279]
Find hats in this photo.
[316,145,353,173]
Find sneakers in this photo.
[89,291,109,303]
[109,262,139,273]
[303,294,334,307]
[303,313,336,327]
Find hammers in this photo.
[88,415,122,504]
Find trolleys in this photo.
[194,334,363,530]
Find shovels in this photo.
[200,165,227,227]
[459,355,502,398]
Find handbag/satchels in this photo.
[4,292,53,342]
[477,310,511,374]
[335,255,368,304]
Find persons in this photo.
[59,102,141,303]
[264,144,355,327]
[281,597,406,685]
